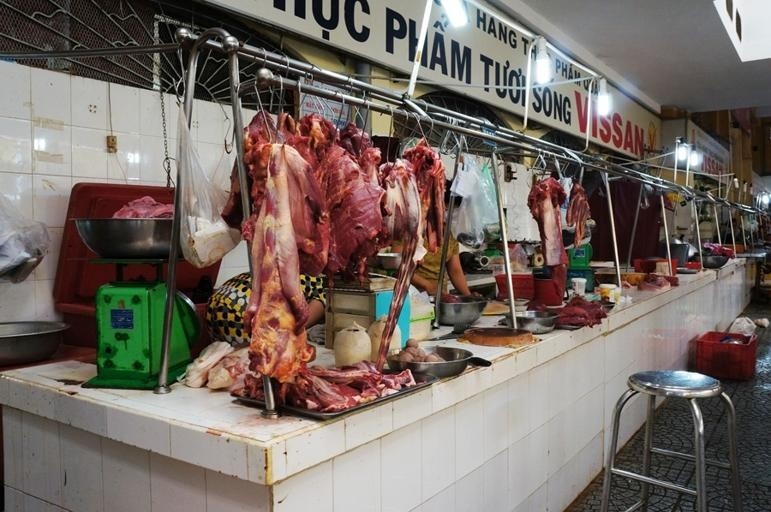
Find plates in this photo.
[675,267,698,274]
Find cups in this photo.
[600,284,619,306]
[570,278,587,297]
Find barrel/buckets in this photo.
[661,244,690,266]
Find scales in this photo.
[67,212,208,391]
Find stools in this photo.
[600,370,743,512]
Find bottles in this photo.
[333,327,372,368]
[368,319,402,364]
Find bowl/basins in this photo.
[431,294,491,326]
[694,255,731,267]
[387,345,475,381]
[0,320,71,366]
[502,311,560,334]
[503,297,531,307]
[366,251,402,269]
[685,262,702,271]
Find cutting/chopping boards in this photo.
[593,272,648,287]
[462,326,535,347]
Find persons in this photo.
[404,178,485,303]
